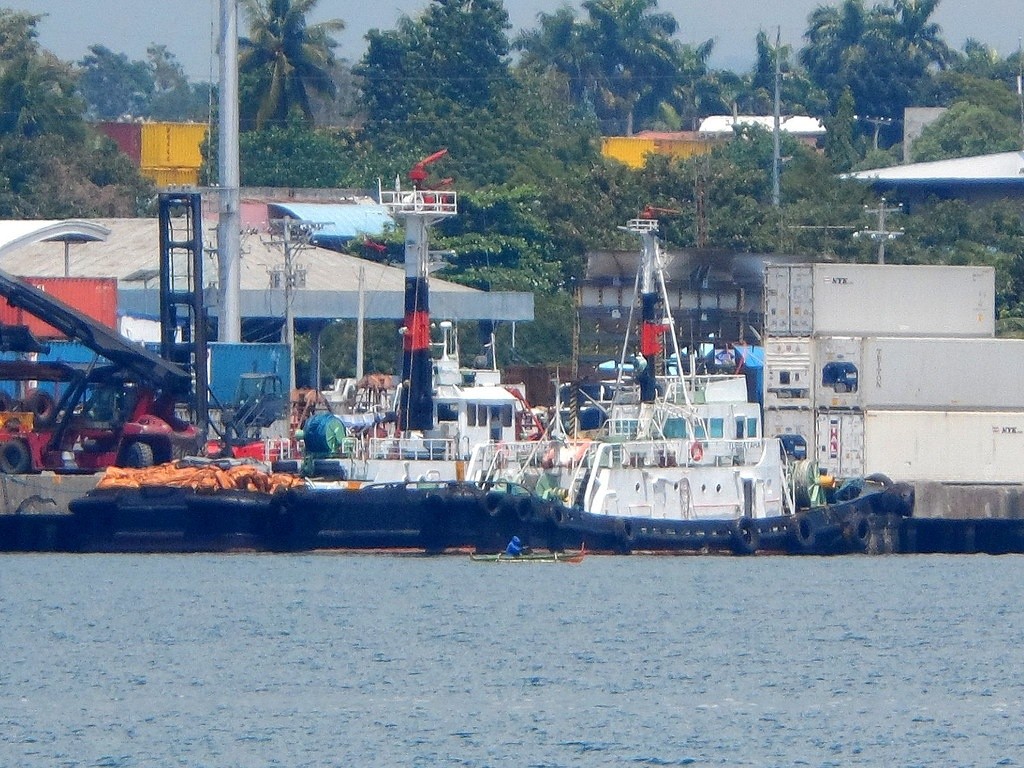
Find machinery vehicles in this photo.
[0,270,204,474]
[157,191,301,462]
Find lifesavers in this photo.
[731,516,760,555]
[851,512,871,545]
[546,503,567,529]
[484,491,504,518]
[691,442,705,462]
[789,513,817,548]
[614,515,637,546]
[901,486,915,517]
[514,497,534,524]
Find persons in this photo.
[541,436,566,474]
[491,416,502,442]
[506,536,526,557]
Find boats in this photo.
[67,147,592,555]
[468,542,585,562]
[265,208,917,555]
